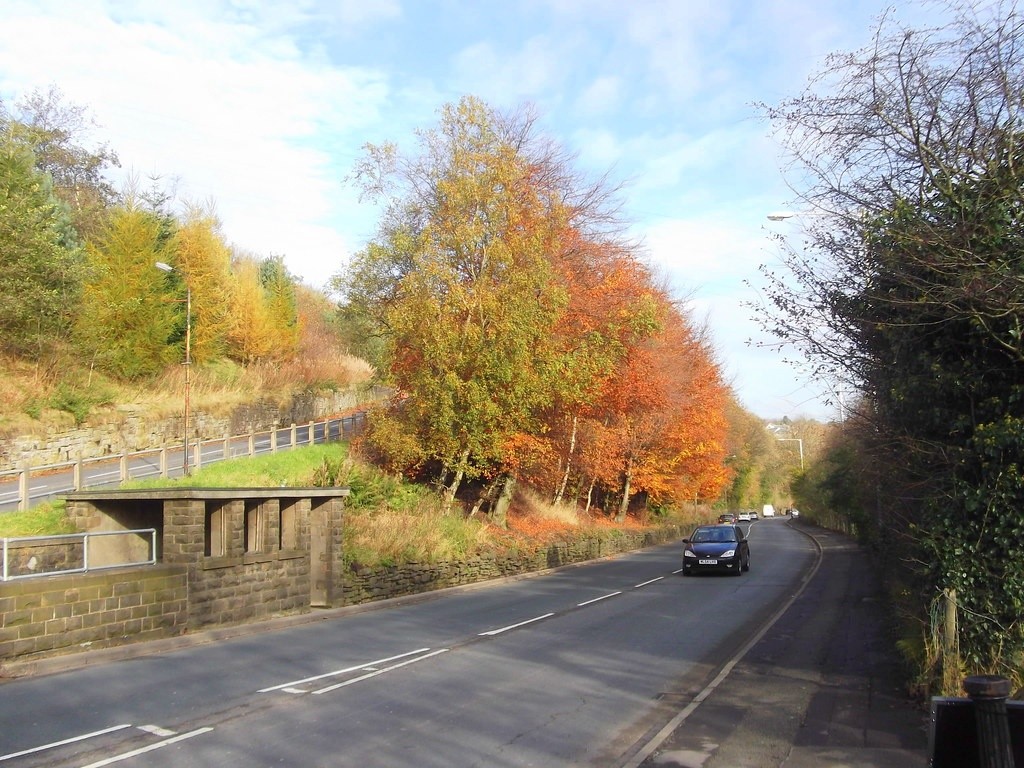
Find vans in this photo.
[763,505,774,517]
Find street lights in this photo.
[155,262,193,477]
[779,437,804,472]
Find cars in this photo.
[681,524,750,576]
[738,512,751,522]
[717,513,737,525]
[748,511,758,520]
